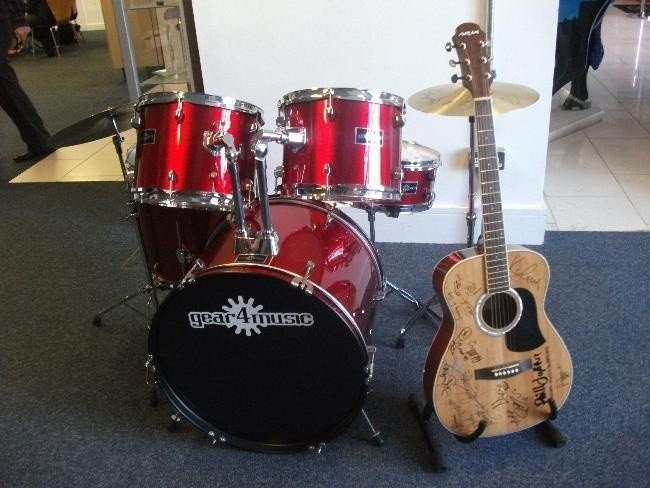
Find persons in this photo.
[1,1,57,164]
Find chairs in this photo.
[30,1,86,62]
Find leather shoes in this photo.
[15,149,53,162]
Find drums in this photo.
[145,194,388,453]
[123,142,263,288]
[275,86,405,203]
[349,140,442,218]
[130,90,265,213]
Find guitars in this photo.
[423,21,574,439]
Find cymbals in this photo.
[45,100,140,150]
[407,81,540,117]
